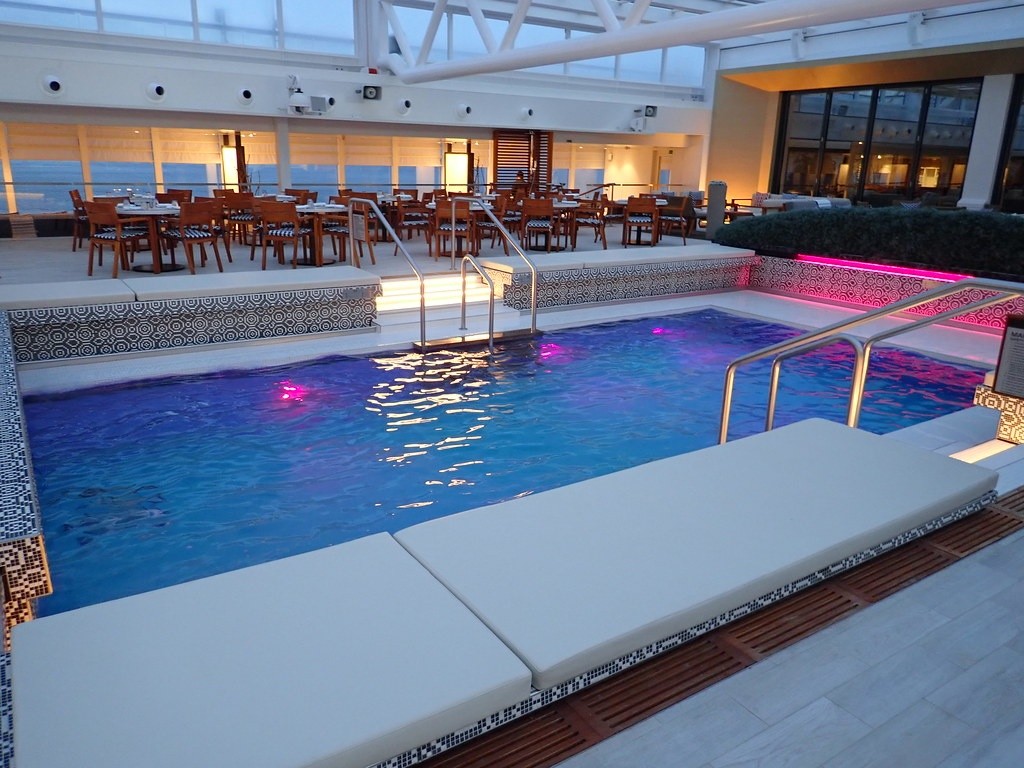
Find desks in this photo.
[617,200,667,245]
[762,200,790,215]
[424,203,494,257]
[116,207,184,272]
[371,197,413,242]
[290,205,349,265]
[517,202,579,252]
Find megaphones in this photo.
[362,85,382,101]
[641,105,658,118]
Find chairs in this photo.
[67,186,852,279]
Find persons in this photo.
[511,170,528,197]
